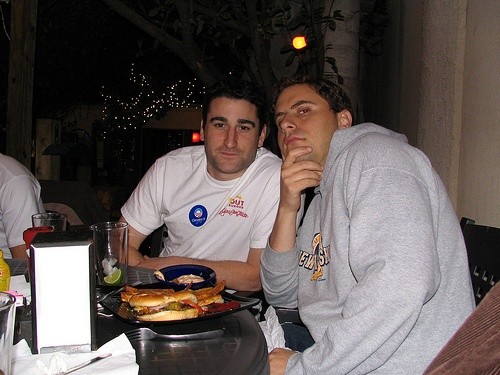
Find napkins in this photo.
[9,333,139,375]
[8,274,31,307]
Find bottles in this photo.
[0,249,11,292]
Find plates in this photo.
[98,281,261,327]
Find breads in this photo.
[121,280,224,306]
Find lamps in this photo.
[42,129,96,158]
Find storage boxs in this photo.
[29,232,97,355]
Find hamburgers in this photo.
[129,292,205,321]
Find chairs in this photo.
[36,180,111,230]
[459,218,500,307]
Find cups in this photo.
[32,212,68,231]
[89,221,130,287]
[0,291,17,375]
[22,225,55,274]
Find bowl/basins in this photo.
[154,264,216,290]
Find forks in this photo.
[125,327,225,342]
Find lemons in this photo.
[104,269,123,284]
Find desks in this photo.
[4,258,270,375]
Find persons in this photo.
[0,153,51,260]
[108,79,306,291]
[257,74,477,375]
